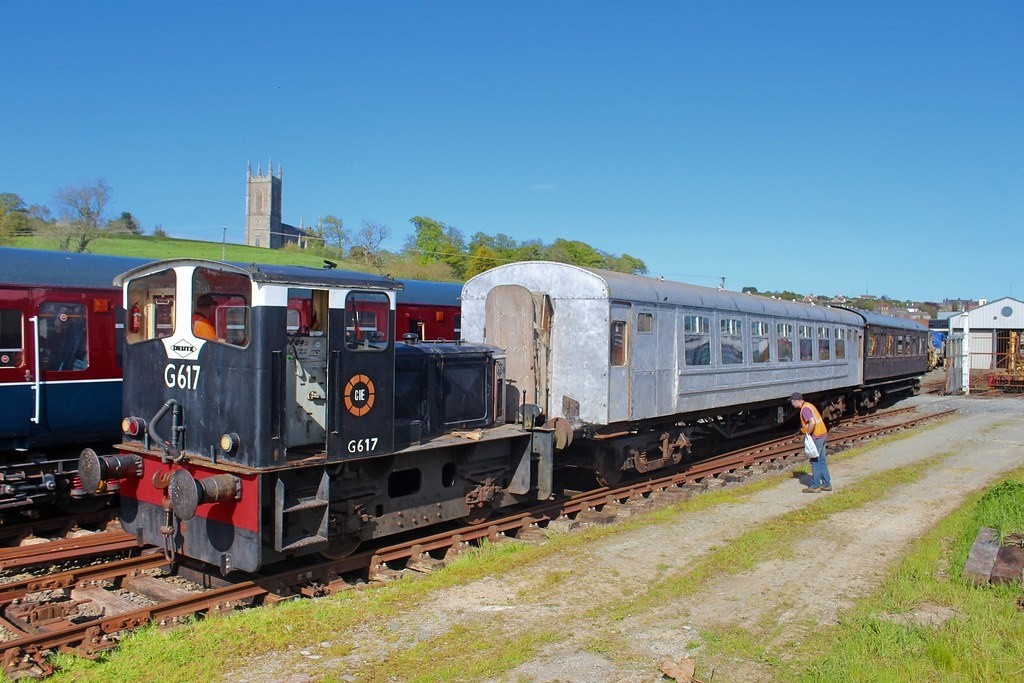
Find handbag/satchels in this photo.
[804,432,819,458]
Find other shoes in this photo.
[803,487,821,493]
[820,486,832,491]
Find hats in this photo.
[788,392,803,401]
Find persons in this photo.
[159,306,216,341]
[788,392,832,492]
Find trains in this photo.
[78,257,929,577]
[0,247,464,516]
